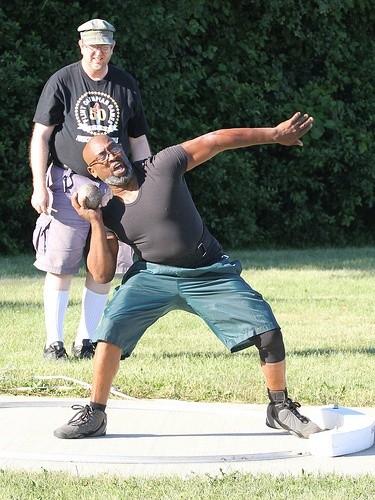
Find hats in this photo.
[77,19,116,45]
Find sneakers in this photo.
[43,341,69,361]
[71,339,95,359]
[53,404,108,439]
[265,398,322,439]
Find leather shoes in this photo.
[52,160,74,173]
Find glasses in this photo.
[84,44,113,53]
[88,143,122,167]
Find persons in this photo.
[31,19,153,361]
[53,112,323,439]
